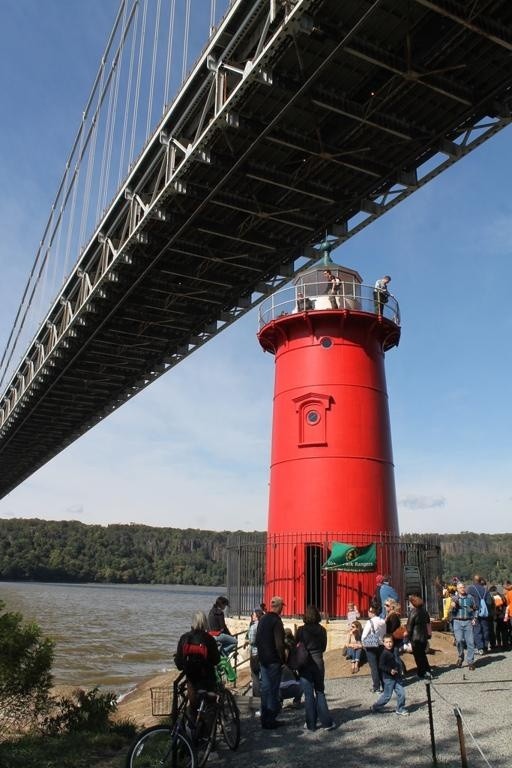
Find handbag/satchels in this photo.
[426,623,432,636]
[363,634,379,648]
[393,625,407,639]
[287,643,309,668]
[343,646,347,655]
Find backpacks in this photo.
[473,585,488,617]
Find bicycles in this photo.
[126,676,219,768]
[194,649,243,767]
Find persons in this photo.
[322,270,339,308]
[297,292,316,312]
[373,276,391,316]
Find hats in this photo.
[270,596,284,606]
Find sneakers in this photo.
[396,710,409,715]
